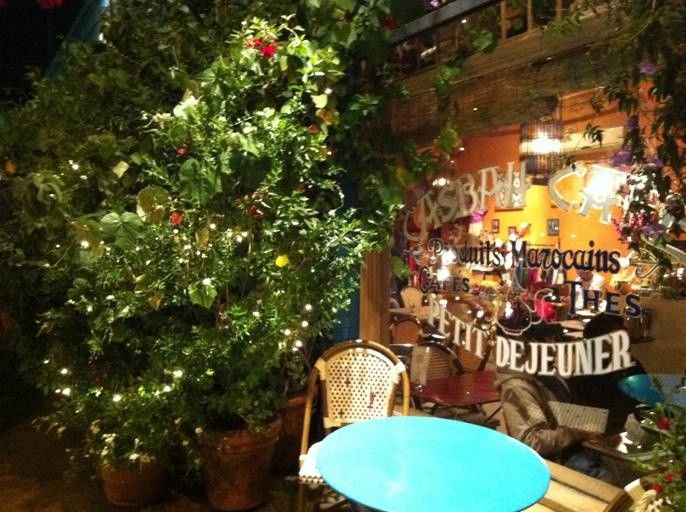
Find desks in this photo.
[315,415,552,512]
[395,370,502,430]
[619,373,685,411]
[582,430,667,488]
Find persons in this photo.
[493,315,625,488]
[494,288,542,338]
[567,310,677,438]
[573,262,607,315]
[499,265,523,290]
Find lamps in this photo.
[519,117,564,186]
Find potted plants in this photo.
[28,14,380,512]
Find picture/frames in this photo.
[547,218,560,236]
[492,219,499,234]
[508,226,516,235]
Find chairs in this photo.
[388,288,502,417]
[298,338,412,511]
[602,472,657,512]
